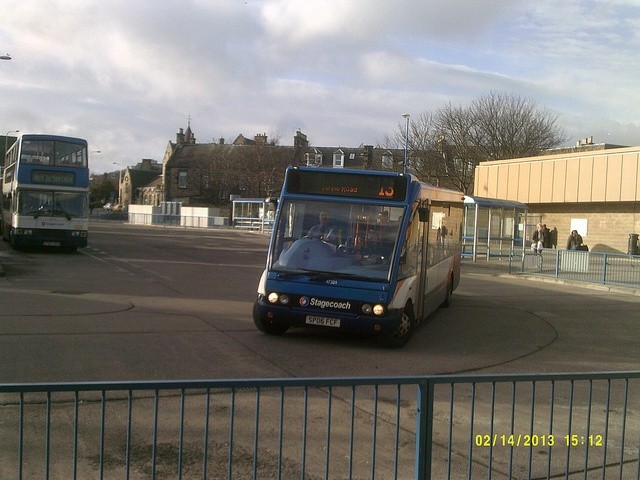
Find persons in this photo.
[566,229,589,251]
[531,223,545,253]
[304,207,337,245]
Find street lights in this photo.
[402,114,410,173]
[5,130,20,152]
[89,151,101,185]
[113,162,121,205]
[0,55,9,61]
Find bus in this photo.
[2,134,93,252]
[252,166,466,349]
